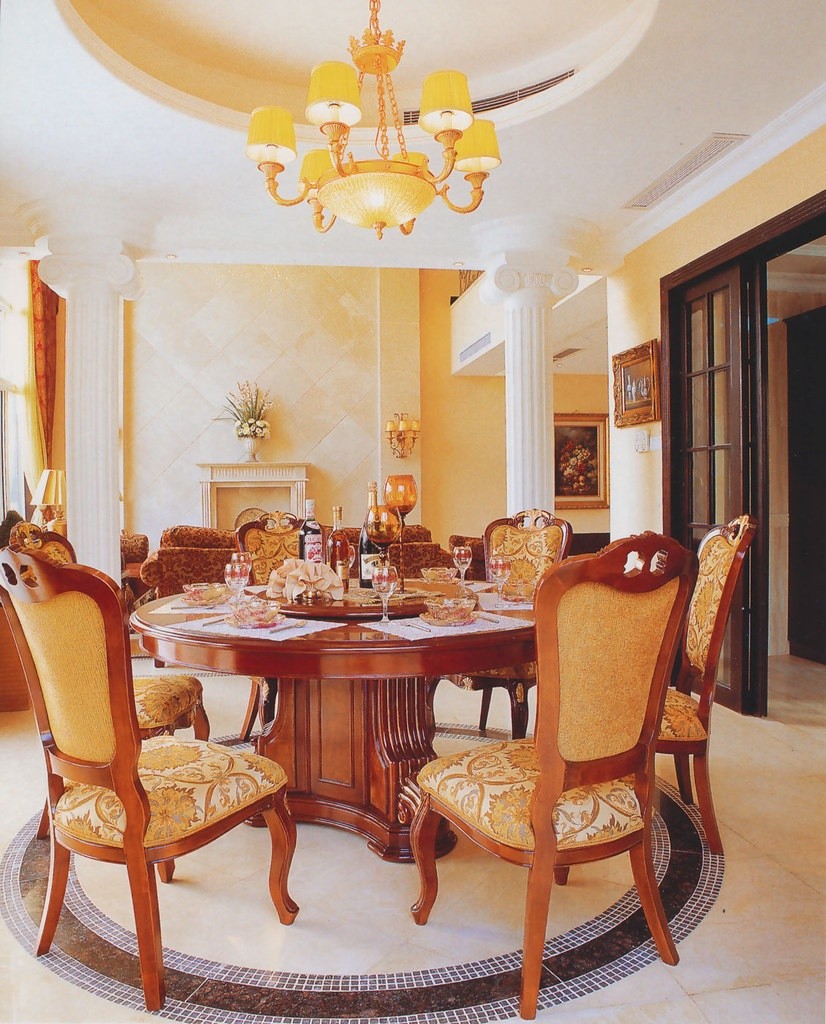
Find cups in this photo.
[349,546,356,570]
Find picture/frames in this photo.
[612,338,661,427]
[551,412,610,509]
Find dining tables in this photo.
[130,579,536,864]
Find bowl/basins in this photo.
[420,567,458,581]
[230,597,281,623]
[424,598,476,620]
[182,583,225,600]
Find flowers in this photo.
[212,380,280,440]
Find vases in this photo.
[239,436,263,462]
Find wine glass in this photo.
[364,505,401,599]
[371,567,399,627]
[383,474,417,595]
[453,546,472,597]
[225,552,252,601]
[488,556,512,611]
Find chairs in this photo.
[0,544,300,1011]
[406,531,700,1021]
[232,508,310,744]
[438,509,575,744]
[652,514,756,855]
[1,510,210,881]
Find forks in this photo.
[400,620,431,632]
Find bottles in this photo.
[358,482,389,588]
[299,500,326,563]
[326,505,350,594]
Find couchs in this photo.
[116,523,485,601]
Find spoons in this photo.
[268,620,306,634]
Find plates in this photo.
[419,612,478,626]
[418,578,460,584]
[224,613,286,629]
[182,595,230,607]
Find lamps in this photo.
[385,413,421,459]
[30,469,67,539]
[246,0,503,243]
